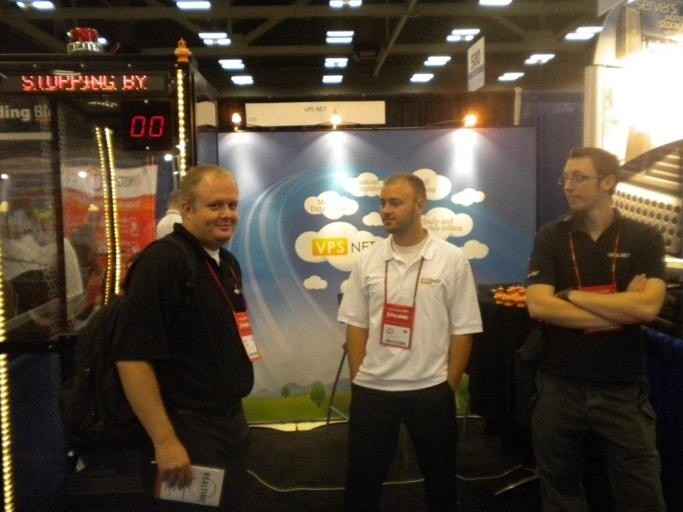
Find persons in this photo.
[525,145,667,512]
[0,195,150,454]
[113,163,255,512]
[336,172,485,512]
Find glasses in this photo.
[558,173,601,187]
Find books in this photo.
[153,465,226,507]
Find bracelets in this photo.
[558,286,577,301]
